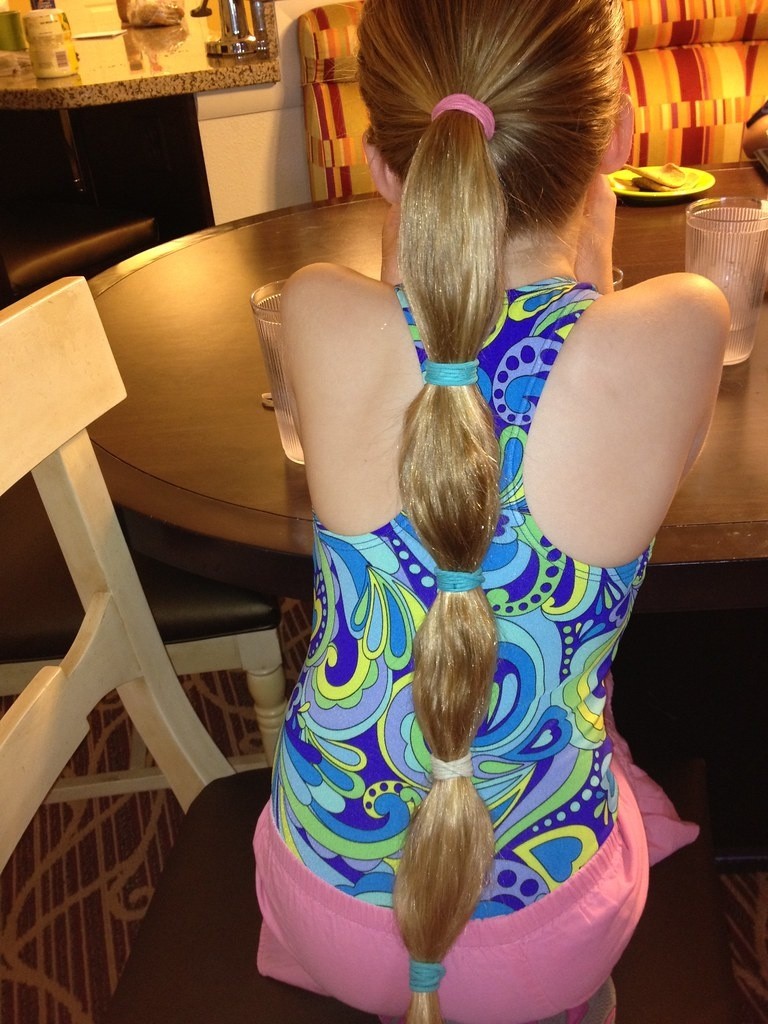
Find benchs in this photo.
[298,0,768,203]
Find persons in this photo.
[252,0,731,1024]
[741,100,768,161]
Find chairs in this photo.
[0,210,383,1024]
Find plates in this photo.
[606,164,716,202]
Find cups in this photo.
[249,278,306,465]
[611,266,624,292]
[21,8,82,80]
[0,10,27,51]
[683,195,768,366]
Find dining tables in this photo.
[75,161,768,869]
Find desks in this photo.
[0,0,281,208]
